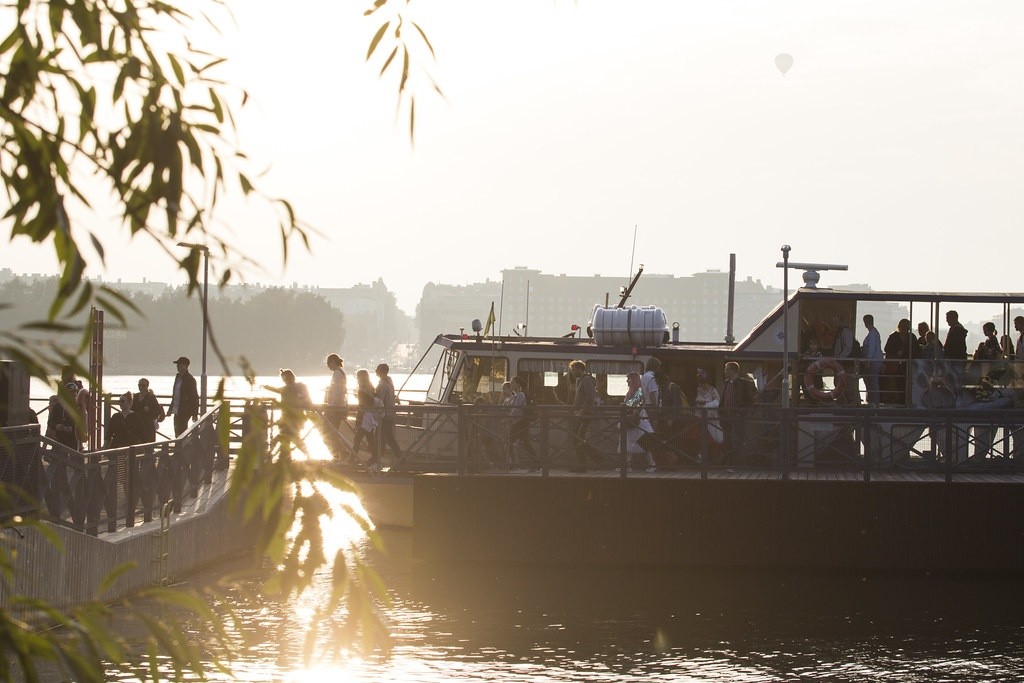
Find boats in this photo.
[309,221,1024,526]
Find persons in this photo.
[641,357,661,408]
[167,356,198,437]
[350,369,374,463]
[374,363,402,464]
[104,393,150,462]
[694,366,723,465]
[501,381,517,470]
[41,379,93,473]
[133,379,160,453]
[718,361,755,474]
[322,352,351,463]
[259,369,312,463]
[659,376,693,426]
[510,376,541,473]
[615,372,658,473]
[800,310,1024,406]
[569,360,607,472]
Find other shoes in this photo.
[726,468,736,472]
[569,464,586,473]
[646,463,657,472]
[504,463,521,471]
[616,465,632,473]
[527,463,542,474]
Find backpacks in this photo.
[359,386,386,419]
[515,391,539,422]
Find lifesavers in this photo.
[804,358,848,402]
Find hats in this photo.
[64,383,81,392]
[173,357,190,366]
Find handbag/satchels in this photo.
[620,407,641,428]
[156,405,165,422]
[847,339,860,359]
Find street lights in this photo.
[781,245,791,482]
[177,240,212,426]
